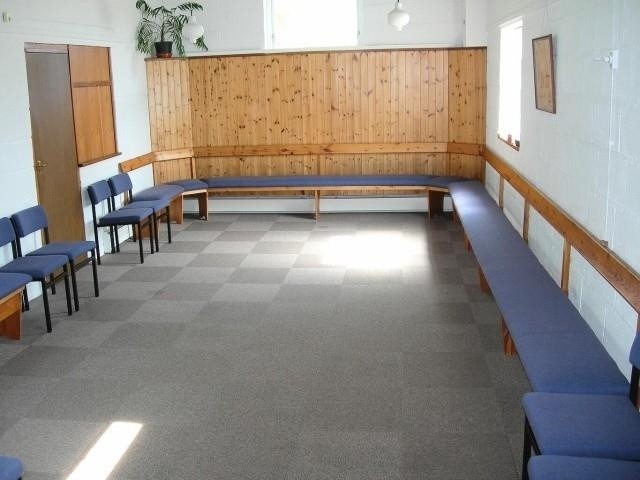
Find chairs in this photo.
[0,172,172,341]
[522,331,640,480]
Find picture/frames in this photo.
[532,34,556,114]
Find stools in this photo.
[163,178,208,224]
[427,176,479,225]
[127,183,183,238]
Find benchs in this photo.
[193,142,448,220]
[448,143,640,394]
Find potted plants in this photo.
[136,0,208,59]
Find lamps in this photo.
[388,0,410,31]
[183,0,204,44]
